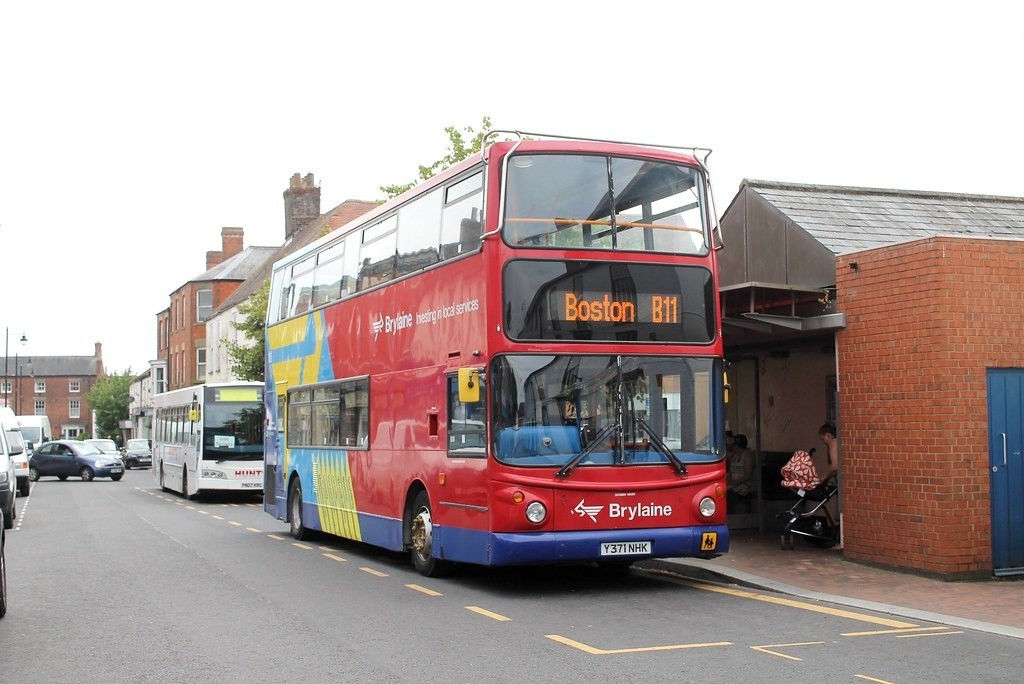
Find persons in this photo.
[725,430,756,513]
[62,447,71,456]
[818,424,844,552]
[113,435,122,450]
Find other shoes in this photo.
[831,544,843,550]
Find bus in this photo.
[152,381,267,500]
[259,130,734,578]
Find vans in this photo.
[0,418,24,530]
[14,415,53,460]
[0,405,35,496]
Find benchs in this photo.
[726,451,796,515]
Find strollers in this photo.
[772,447,841,551]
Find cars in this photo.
[26,439,126,484]
[120,439,153,469]
[0,505,8,617]
[83,438,124,462]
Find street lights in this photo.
[5,327,29,408]
[20,366,37,415]
[14,352,33,415]
